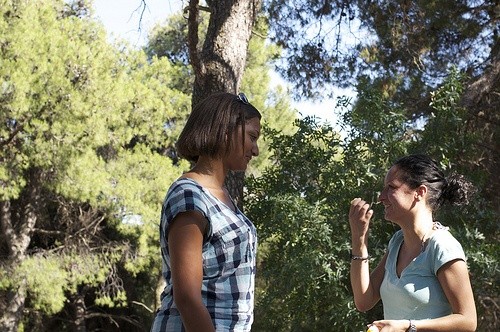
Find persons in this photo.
[149,92,262,332]
[348,154,478,332]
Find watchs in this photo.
[407,320,417,332]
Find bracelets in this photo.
[352,255,368,260]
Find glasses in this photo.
[235,92,249,104]
